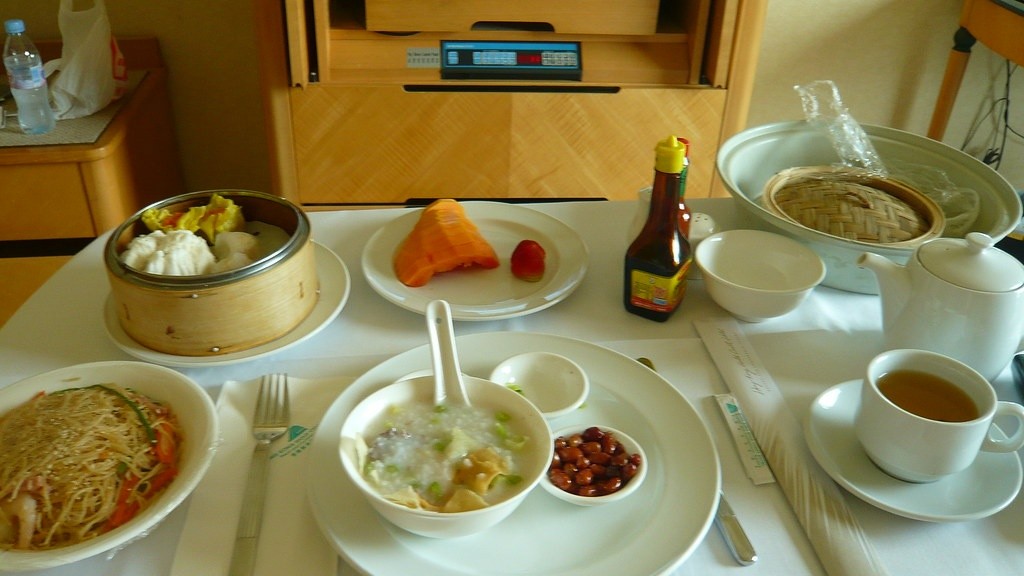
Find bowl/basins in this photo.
[339,371,554,539]
[716,120,1023,293]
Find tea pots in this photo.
[857,233,1024,403]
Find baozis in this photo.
[119,193,259,277]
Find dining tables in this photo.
[0,197,1024,576]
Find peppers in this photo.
[98,419,182,532]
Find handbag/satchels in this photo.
[43,0,131,117]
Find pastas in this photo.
[0,385,178,553]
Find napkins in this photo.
[171,374,353,576]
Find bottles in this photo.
[623,136,694,319]
[2,20,57,136]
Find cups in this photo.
[851,349,1024,483]
[694,230,826,323]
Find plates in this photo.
[309,332,723,576]
[0,361,218,574]
[360,200,589,321]
[102,238,352,366]
[488,351,589,421]
[804,379,1023,521]
[536,422,648,505]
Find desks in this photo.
[928,1,1023,141]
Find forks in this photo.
[228,374,293,576]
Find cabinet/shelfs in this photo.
[0,34,179,329]
[252,0,768,206]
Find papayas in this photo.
[395,197,499,287]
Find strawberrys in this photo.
[512,239,547,282]
